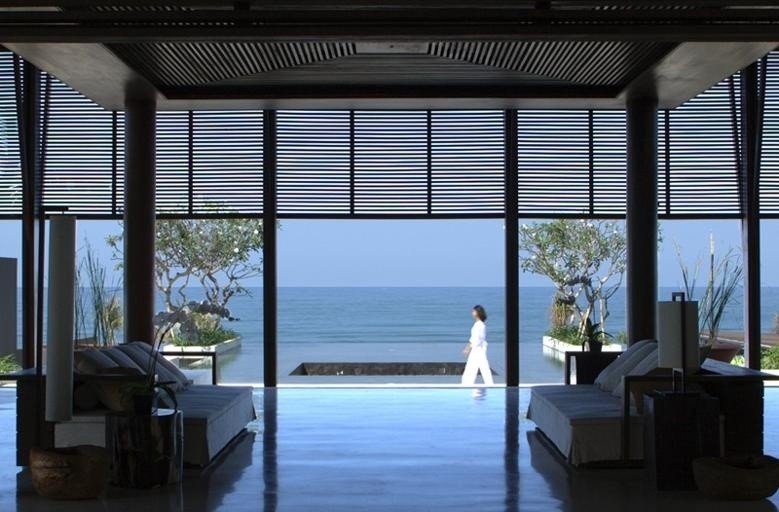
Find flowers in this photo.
[120,299,233,409]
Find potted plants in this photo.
[674,234,743,364]
[579,317,614,352]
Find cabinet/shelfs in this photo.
[641,387,722,491]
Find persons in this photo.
[462,305,493,383]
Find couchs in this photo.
[72,341,257,469]
[527,340,779,469]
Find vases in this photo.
[133,393,158,414]
